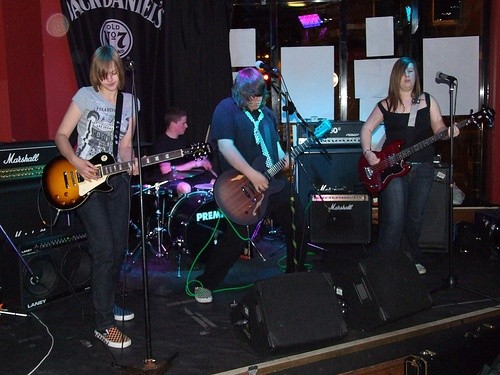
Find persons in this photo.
[194,68,309,303]
[151,109,216,195]
[361,57,460,275]
[54,45,140,348]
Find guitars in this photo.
[358,103,496,194]
[213,119,332,227]
[40,139,212,213]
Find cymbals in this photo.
[151,169,205,186]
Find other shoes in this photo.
[194,287,212,303]
[415,263,427,275]
[96,326,132,348]
[113,306,136,320]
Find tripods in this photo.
[128,178,181,262]
[429,80,500,303]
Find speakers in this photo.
[0,178,93,314]
[310,190,371,244]
[249,270,348,355]
[295,146,373,262]
[418,164,451,253]
[342,251,434,333]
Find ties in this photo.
[246,109,274,169]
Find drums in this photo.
[167,189,230,263]
[193,183,214,193]
[131,184,165,212]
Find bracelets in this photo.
[365,150,369,154]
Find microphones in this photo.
[125,61,135,70]
[254,60,274,74]
[436,72,457,82]
